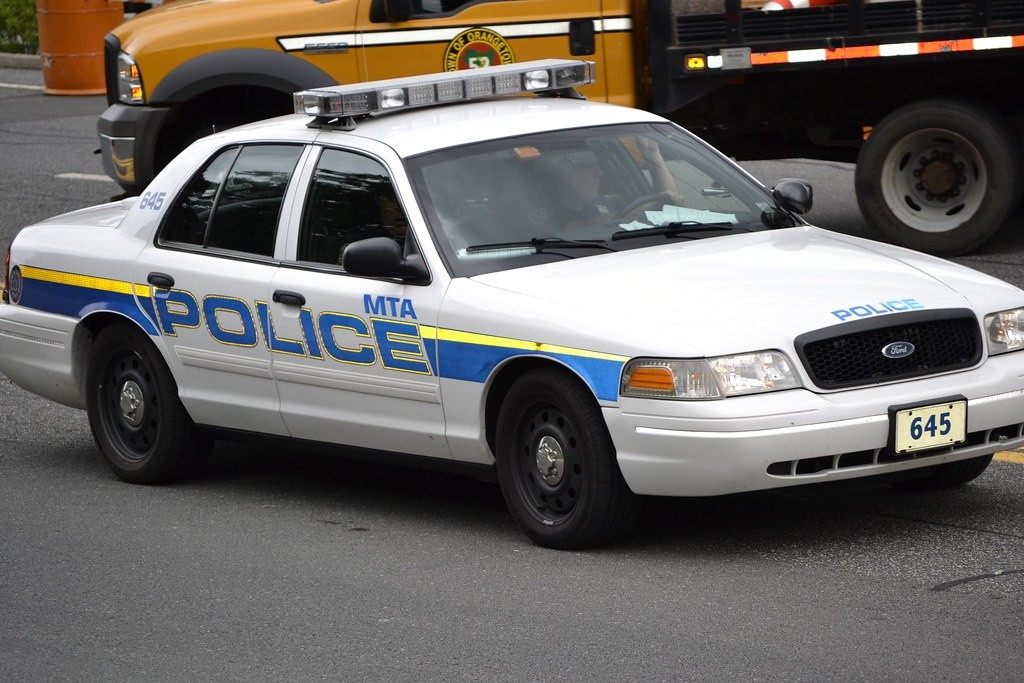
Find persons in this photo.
[338,185,467,267]
[540,135,680,235]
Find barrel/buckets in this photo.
[37,0,124,95]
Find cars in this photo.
[0,82,1024,545]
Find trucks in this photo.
[99,0,1024,258]
